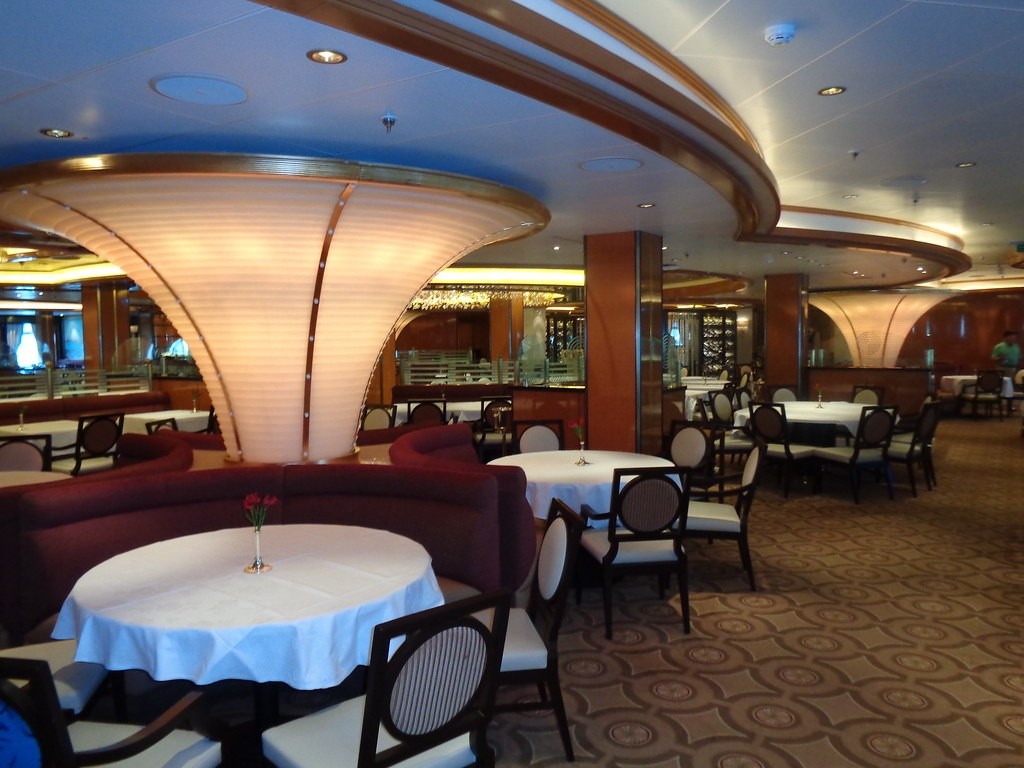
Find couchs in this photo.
[0,433,193,633]
[0,390,172,451]
[389,424,484,465]
[15,466,538,600]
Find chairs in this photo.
[0,405,218,475]
[960,370,1003,422]
[262,590,509,768]
[0,656,220,768]
[362,394,514,442]
[1001,370,1024,417]
[516,363,940,629]
[473,498,584,762]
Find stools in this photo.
[0,640,126,725]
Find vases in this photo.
[817,396,822,408]
[243,494,278,574]
[250,531,264,572]
[568,417,586,465]
[576,442,586,465]
[816,384,828,408]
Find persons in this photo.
[991,331,1023,411]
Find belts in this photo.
[1000,365,1017,368]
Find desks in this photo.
[118,409,211,436]
[486,450,675,587]
[0,471,74,488]
[931,375,982,415]
[733,401,897,485]
[0,420,89,457]
[393,401,512,425]
[664,372,729,421]
[72,524,433,768]
